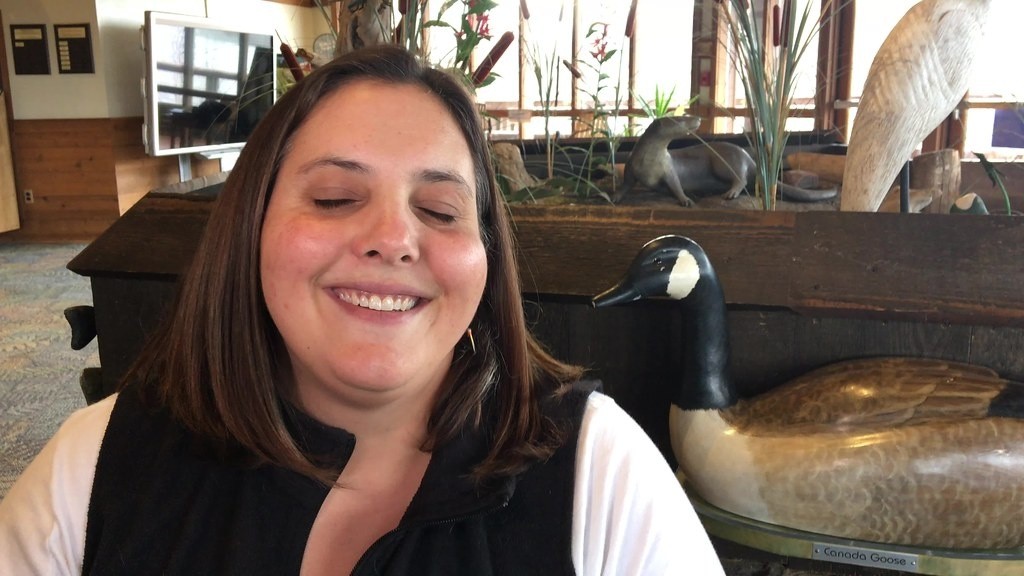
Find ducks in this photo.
[590,235,1024,553]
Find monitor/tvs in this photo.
[144,10,278,155]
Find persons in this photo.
[0,45,727,576]
